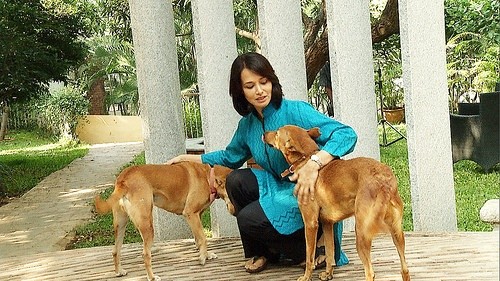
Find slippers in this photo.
[313,246,326,269]
[246,254,268,272]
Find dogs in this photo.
[262,124,411,281]
[92,161,237,281]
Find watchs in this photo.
[309,154,324,170]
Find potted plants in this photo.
[381,94,404,125]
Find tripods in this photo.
[375,60,406,147]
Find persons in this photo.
[160,52,357,274]
